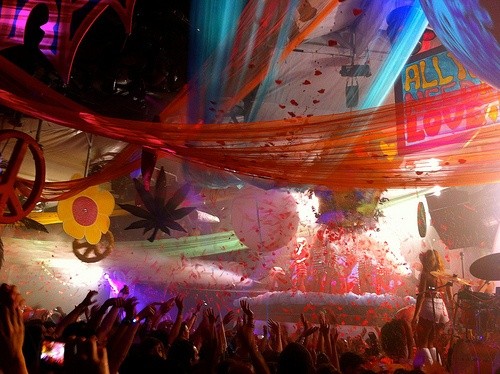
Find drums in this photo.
[461,308,494,330]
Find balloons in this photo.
[229,184,300,253]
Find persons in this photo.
[412,249,450,368]
[0,281,382,374]
[270,230,377,296]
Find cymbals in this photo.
[470,253,500,281]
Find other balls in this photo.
[231,187,298,251]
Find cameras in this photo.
[38,338,78,371]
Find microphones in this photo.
[447,280,453,286]
[460,252,464,256]
